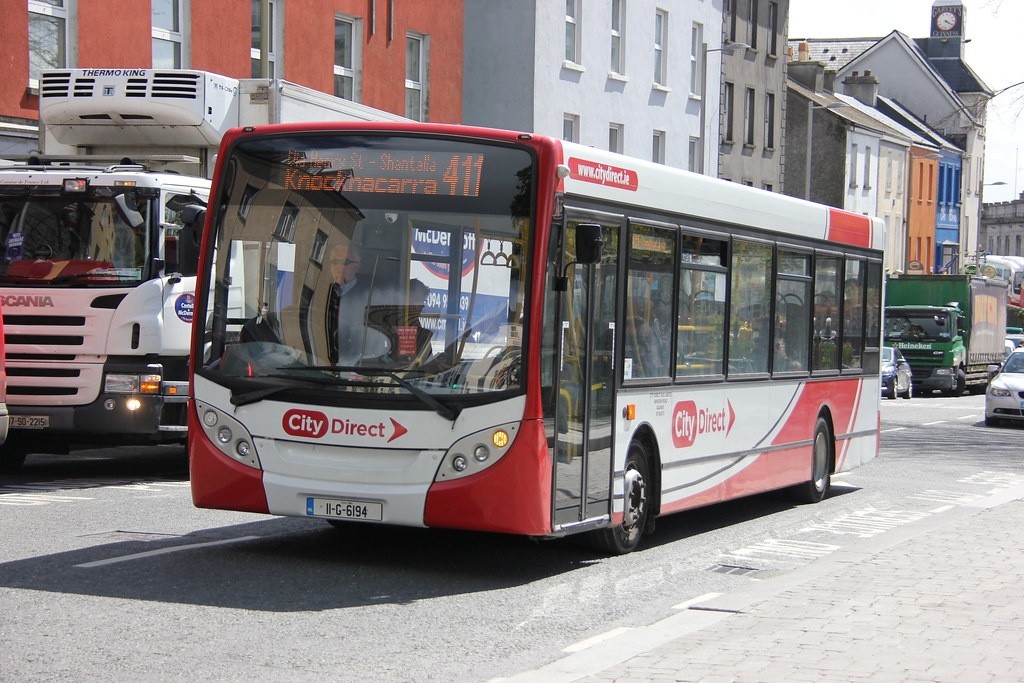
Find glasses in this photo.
[329,258,358,266]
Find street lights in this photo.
[973,180,1009,276]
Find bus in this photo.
[179,122,890,555]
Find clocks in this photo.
[930,6,963,35]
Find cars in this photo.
[985,333,1024,425]
[881,347,913,399]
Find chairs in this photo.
[624,286,842,382]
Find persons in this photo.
[311,243,384,325]
[816,278,874,336]
[408,278,429,330]
[755,332,800,370]
[655,275,689,318]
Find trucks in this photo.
[879,272,1007,398]
[1,68,566,480]
